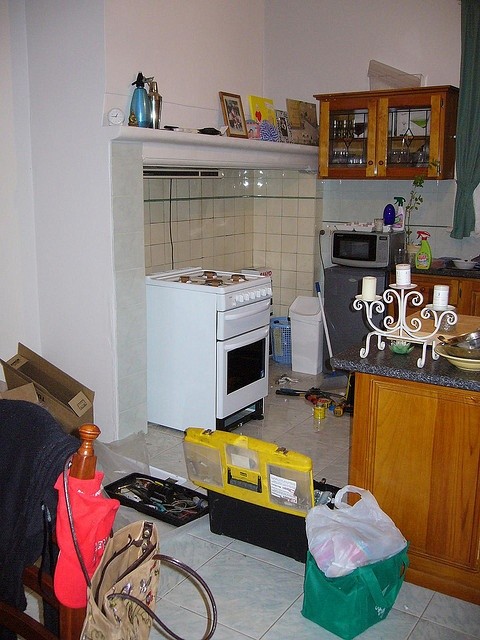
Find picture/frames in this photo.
[219,91,248,139]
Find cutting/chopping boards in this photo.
[387,310,480,346]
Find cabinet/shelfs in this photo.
[318,85,459,180]
[347,372,480,607]
[388,274,480,323]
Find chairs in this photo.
[0,399,101,640]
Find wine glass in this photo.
[330,118,356,140]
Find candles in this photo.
[433,285,449,307]
[396,264,411,287]
[362,276,376,299]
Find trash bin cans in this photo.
[289,295,324,375]
[270,317,291,364]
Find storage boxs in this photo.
[0,341,95,434]
[182,427,341,566]
[0,379,40,404]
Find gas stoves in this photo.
[145,267,272,311]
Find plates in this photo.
[442,329,480,357]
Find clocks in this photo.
[107,108,125,126]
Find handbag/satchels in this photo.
[300,541,410,640]
[63,454,217,640]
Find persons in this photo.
[229,109,236,127]
[280,117,288,136]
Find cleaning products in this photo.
[415,231,431,270]
[392,197,406,231]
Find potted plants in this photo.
[405,159,435,269]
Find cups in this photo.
[374,219,383,232]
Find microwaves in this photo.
[331,231,405,270]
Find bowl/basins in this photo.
[453,260,477,270]
[435,343,480,372]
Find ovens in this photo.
[145,298,271,434]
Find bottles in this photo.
[312,401,326,433]
